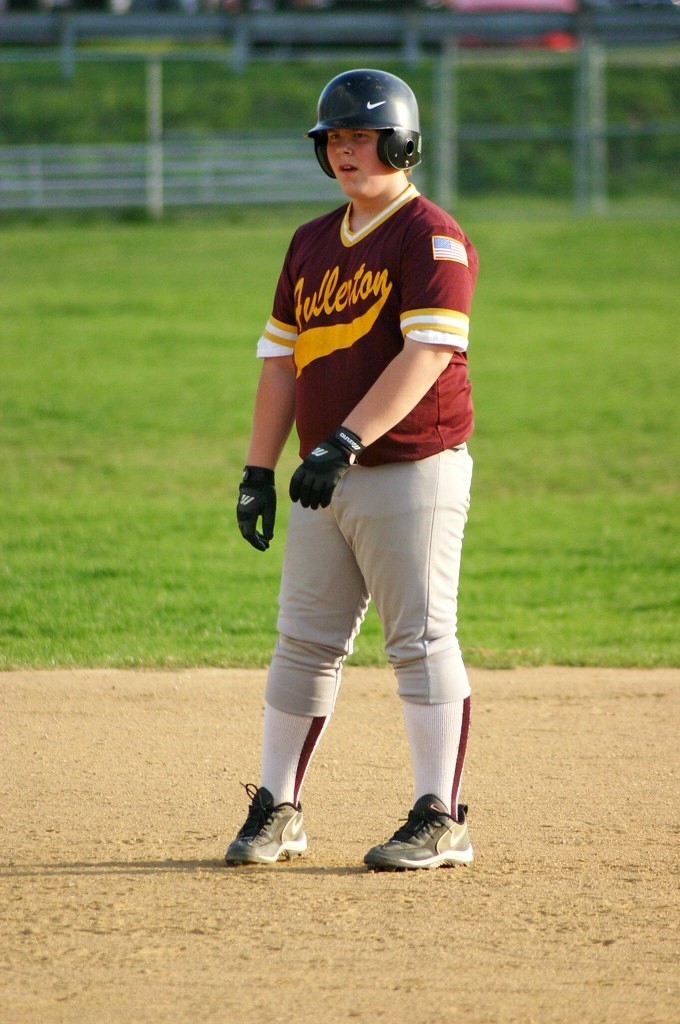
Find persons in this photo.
[224,66,477,870]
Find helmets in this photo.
[304,67,422,179]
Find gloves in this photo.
[289,426,365,511]
[236,465,277,552]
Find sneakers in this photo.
[225,781,307,865]
[363,794,474,870]
